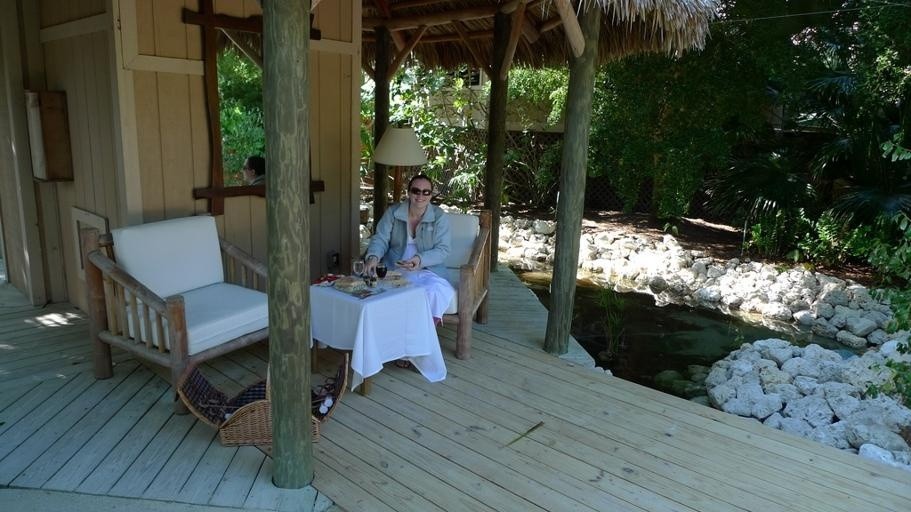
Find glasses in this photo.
[409,188,432,196]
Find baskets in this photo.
[176,352,351,446]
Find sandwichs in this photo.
[395,260,413,268]
[336,278,364,293]
[373,269,401,282]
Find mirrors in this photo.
[179,0,324,216]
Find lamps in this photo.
[372,121,429,202]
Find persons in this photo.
[242,156,265,185]
[362,176,453,368]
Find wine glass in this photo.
[353,259,365,279]
[376,263,387,293]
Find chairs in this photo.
[81,213,268,415]
[433,210,493,358]
